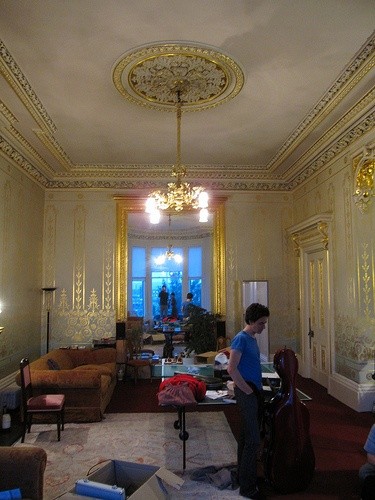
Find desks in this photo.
[159,356,313,471]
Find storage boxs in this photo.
[55,460,185,500]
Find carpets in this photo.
[11,411,255,500]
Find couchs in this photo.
[15,349,117,424]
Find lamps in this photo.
[145,79,209,225]
[42,288,57,354]
[155,216,185,265]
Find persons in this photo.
[159,285,169,314]
[182,293,193,317]
[169,293,178,317]
[358,422,375,485]
[228,302,270,500]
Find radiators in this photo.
[0,369,22,432]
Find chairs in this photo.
[19,357,65,443]
[125,349,155,384]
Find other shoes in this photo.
[240,486,266,500]
